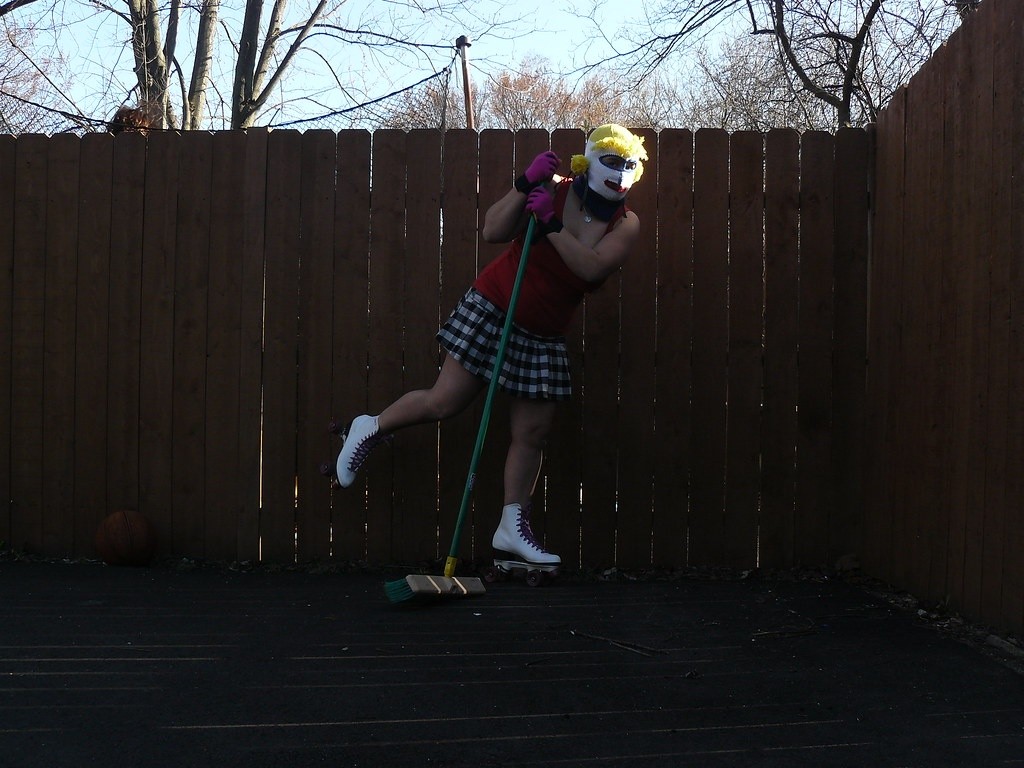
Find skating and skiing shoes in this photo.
[320,413,397,491]
[490,502,560,588]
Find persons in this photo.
[112,107,148,136]
[320,125,649,586]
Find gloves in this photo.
[524,185,564,236]
[515,150,561,196]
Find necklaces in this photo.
[583,207,592,223]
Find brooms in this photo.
[384,181,545,607]
